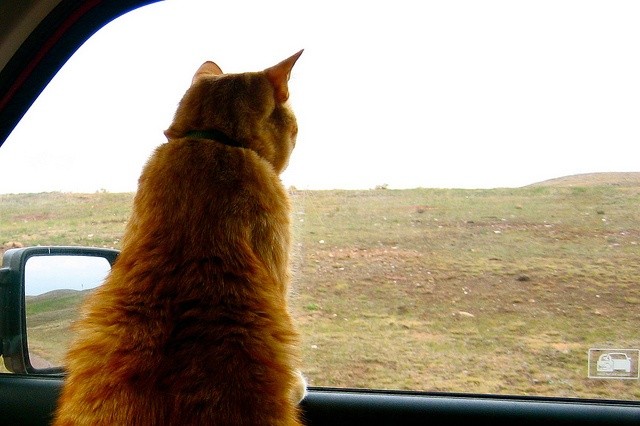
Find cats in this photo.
[42,48,302,426]
